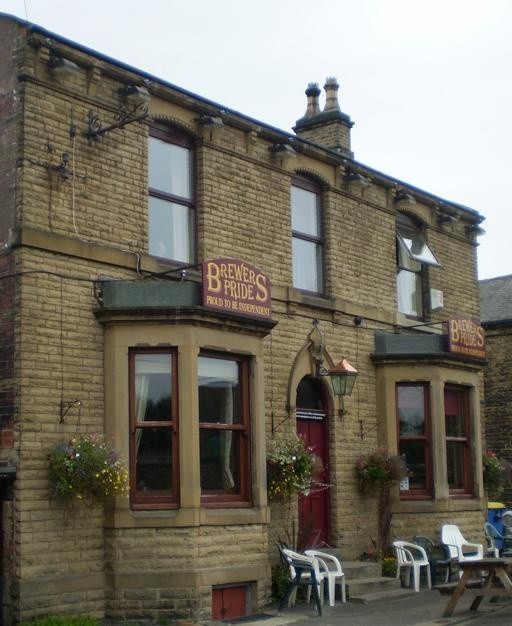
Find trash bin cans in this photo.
[487,501,507,552]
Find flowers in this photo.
[263,426,333,505]
[354,444,414,489]
[48,424,132,511]
[482,449,507,501]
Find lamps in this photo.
[394,193,486,238]
[268,143,299,161]
[117,85,151,106]
[330,356,358,417]
[344,172,368,189]
[200,108,226,130]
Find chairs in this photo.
[278,546,347,616]
[391,522,511,593]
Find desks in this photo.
[442,556,512,618]
